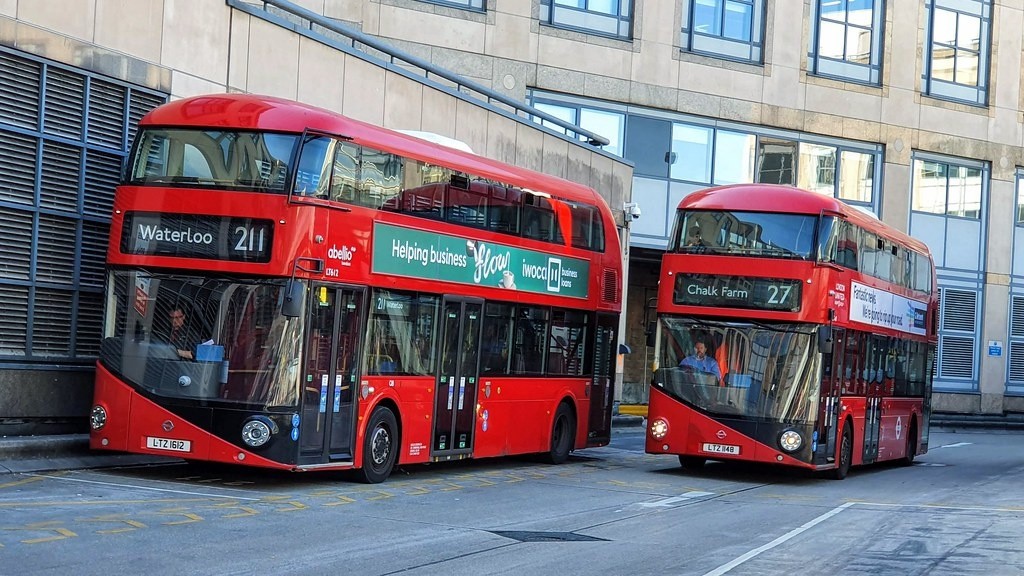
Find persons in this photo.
[684,226,717,253]
[156,307,203,362]
[481,323,505,355]
[675,341,723,384]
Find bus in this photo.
[89,95,623,484]
[645,181,940,479]
